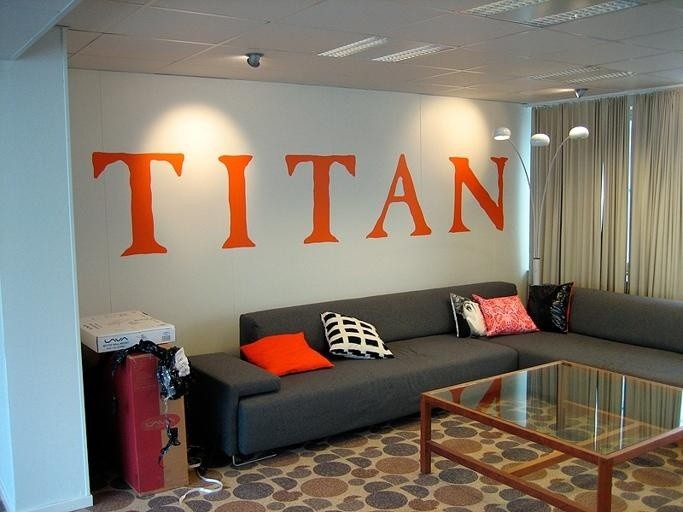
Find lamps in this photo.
[493,126,589,262]
[247,53,263,67]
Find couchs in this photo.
[189,282,683,467]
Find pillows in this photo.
[239,332,334,377]
[449,283,573,337]
[321,312,396,359]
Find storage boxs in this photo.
[110,344,190,496]
